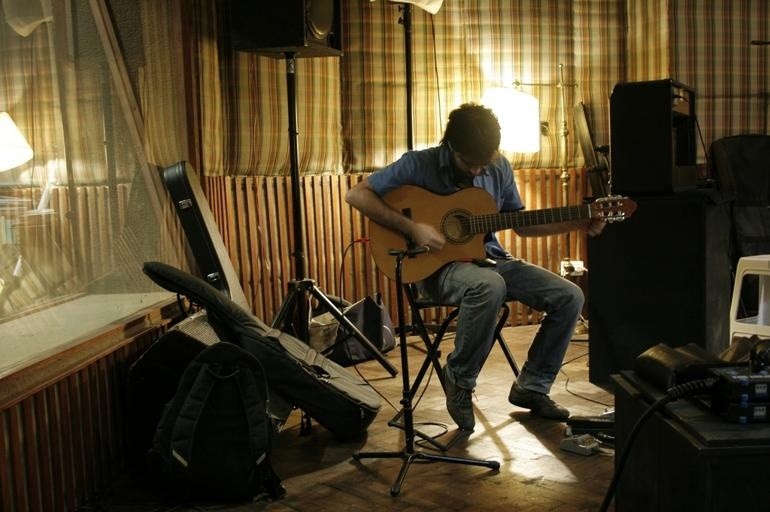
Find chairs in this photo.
[385,282,522,426]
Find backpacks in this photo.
[132,342,286,506]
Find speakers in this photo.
[585,184,732,385]
[609,78,700,197]
[230,0,344,58]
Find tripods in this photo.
[270,56,399,378]
[352,254,500,497]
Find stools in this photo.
[729,254,770,348]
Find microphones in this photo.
[389,245,430,256]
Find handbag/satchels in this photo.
[309,293,397,369]
[634,343,726,393]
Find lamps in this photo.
[0,110,35,172]
[480,61,591,341]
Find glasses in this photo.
[454,151,491,166]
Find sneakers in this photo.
[443,365,474,430]
[509,381,570,420]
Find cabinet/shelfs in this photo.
[603,369,770,512]
[0,290,203,512]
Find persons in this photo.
[343,103,608,430]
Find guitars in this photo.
[369,185,638,284]
